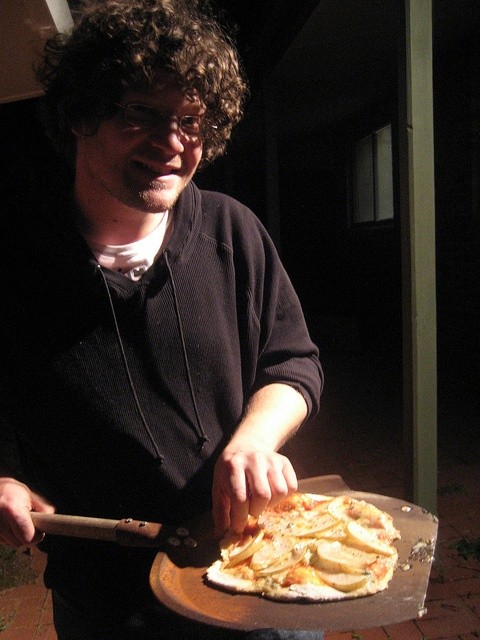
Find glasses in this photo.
[115,98,218,141]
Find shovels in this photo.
[30,475,438,632]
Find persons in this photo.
[0,0,325,638]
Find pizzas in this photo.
[202,493,401,604]
